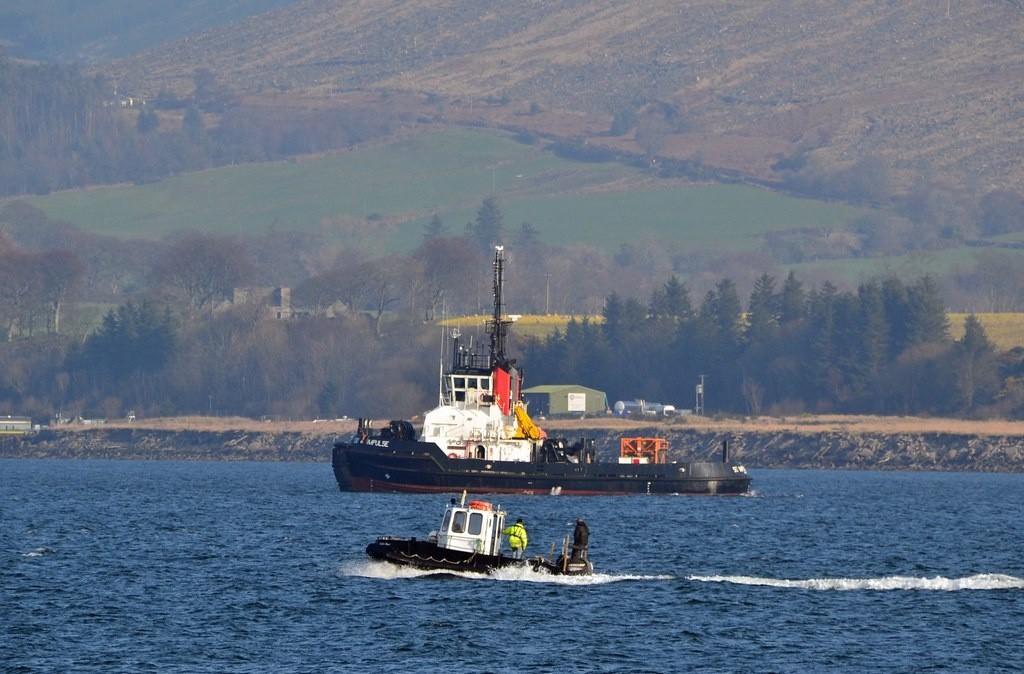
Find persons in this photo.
[452,524,463,533]
[499,518,528,561]
[570,517,590,559]
[585,441,603,464]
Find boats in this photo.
[365,490,593,580]
[331,244,754,498]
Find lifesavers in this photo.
[448,453,458,460]
